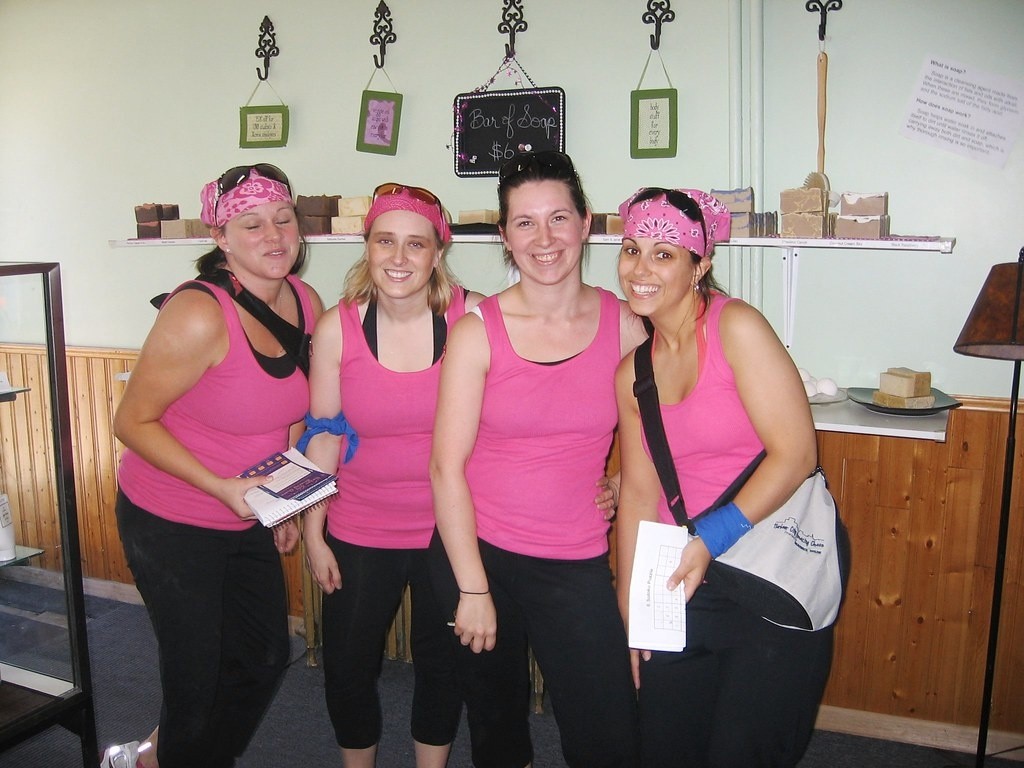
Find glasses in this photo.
[371,183,444,222]
[215,163,292,227]
[628,188,708,254]
[499,151,581,194]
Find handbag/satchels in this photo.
[692,459,853,653]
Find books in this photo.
[234,447,338,529]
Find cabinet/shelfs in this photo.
[0,259,98,768]
[107,234,955,442]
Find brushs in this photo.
[802,54,832,195]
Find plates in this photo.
[847,386,963,417]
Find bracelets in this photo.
[693,502,754,560]
[459,589,490,595]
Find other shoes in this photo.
[100,741,144,768]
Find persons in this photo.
[304,182,488,768]
[428,151,650,768]
[614,188,850,768]
[99,163,327,768]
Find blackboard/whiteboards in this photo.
[453,88,566,177]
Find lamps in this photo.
[953,245,1024,768]
